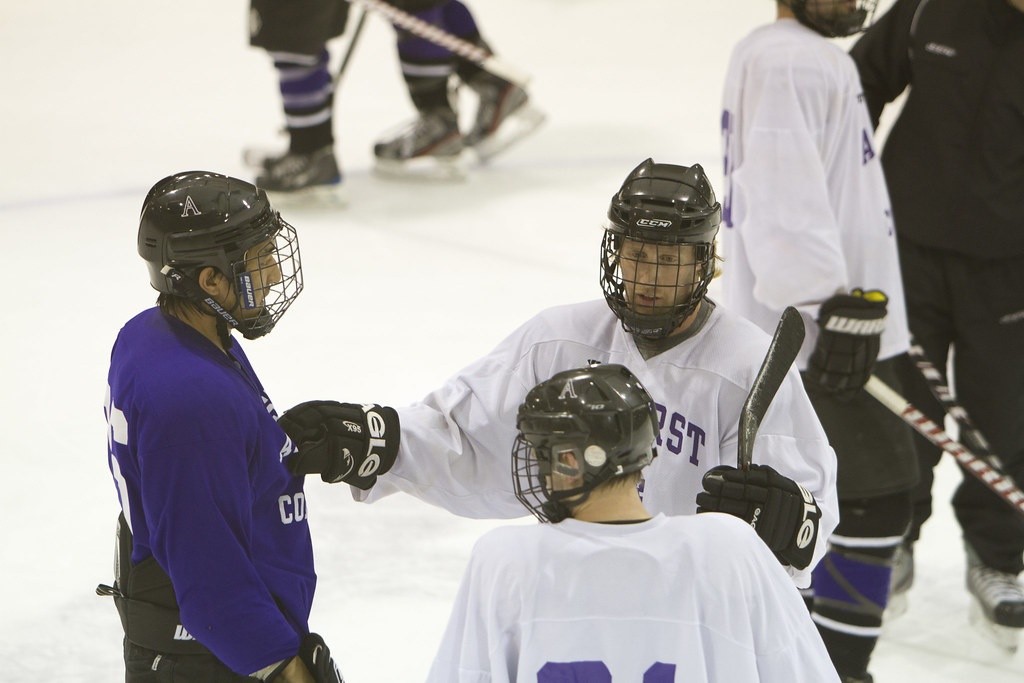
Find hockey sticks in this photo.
[863,374,1024,511]
[905,329,1015,485]
[737,306,806,469]
[278,6,370,135]
[350,0,559,94]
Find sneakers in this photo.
[463,76,541,160]
[965,536,1024,646]
[373,108,476,181]
[888,540,914,613]
[257,140,345,205]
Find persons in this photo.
[245,0,551,198]
[277,158,841,590]
[716,0,927,683]
[427,364,842,683]
[846,0,1024,652]
[102,171,346,683]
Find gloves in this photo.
[806,289,893,409]
[697,463,822,571]
[275,400,400,491]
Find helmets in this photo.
[605,158,721,341]
[791,0,868,37]
[136,172,283,341]
[516,363,660,524]
